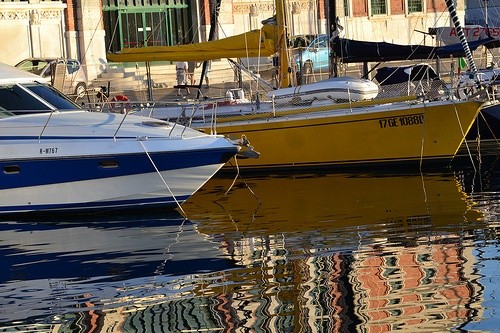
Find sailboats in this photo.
[413,0,500,144]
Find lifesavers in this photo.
[108,95,132,114]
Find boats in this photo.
[0,63,259,224]
[76,0,489,174]
[262,75,378,102]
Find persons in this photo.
[175,61,212,96]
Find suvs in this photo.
[14,56,87,98]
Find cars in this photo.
[275,31,338,73]
